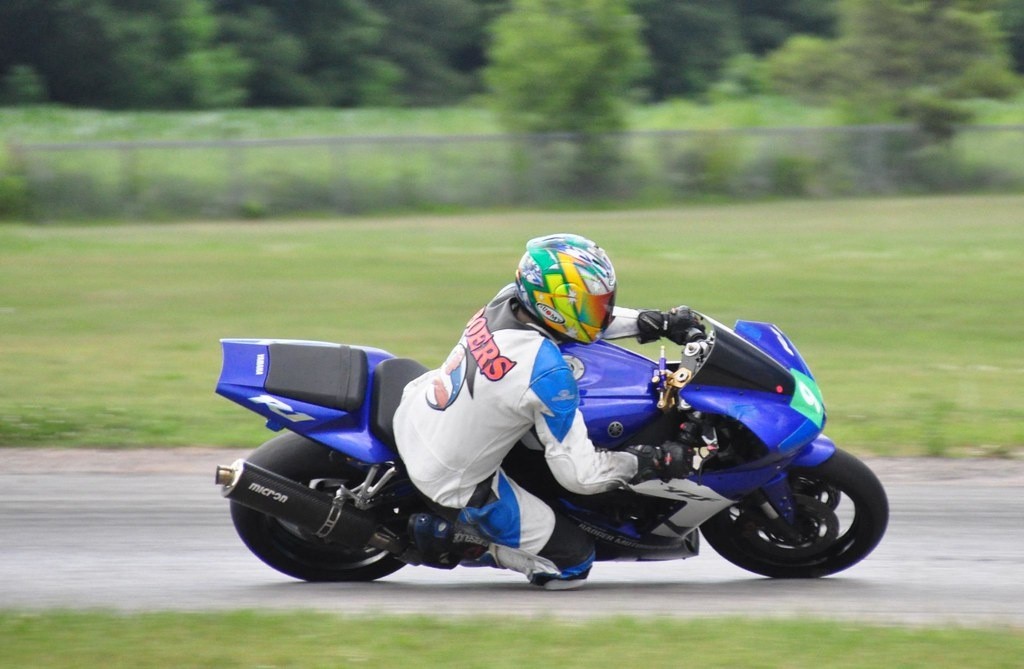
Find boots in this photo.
[407,513,504,571]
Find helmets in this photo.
[515,234,617,345]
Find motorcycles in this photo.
[215,303,891,587]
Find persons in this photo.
[390,233,701,590]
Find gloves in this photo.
[636,305,706,344]
[626,440,696,486]
